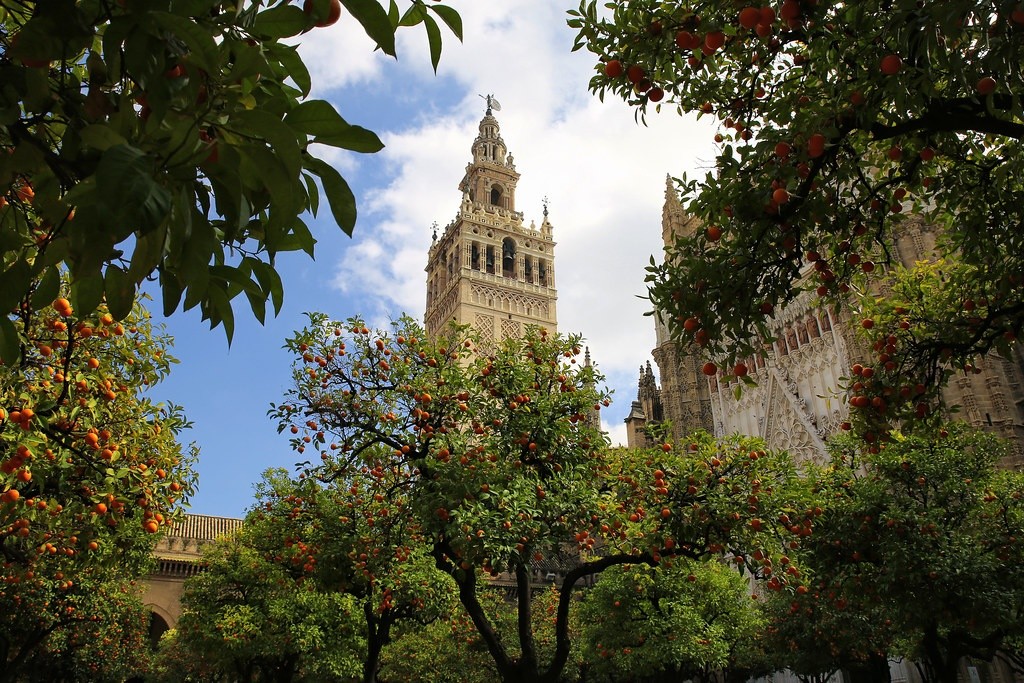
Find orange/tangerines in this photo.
[0,38,260,683]
[248,0,1024,671]
